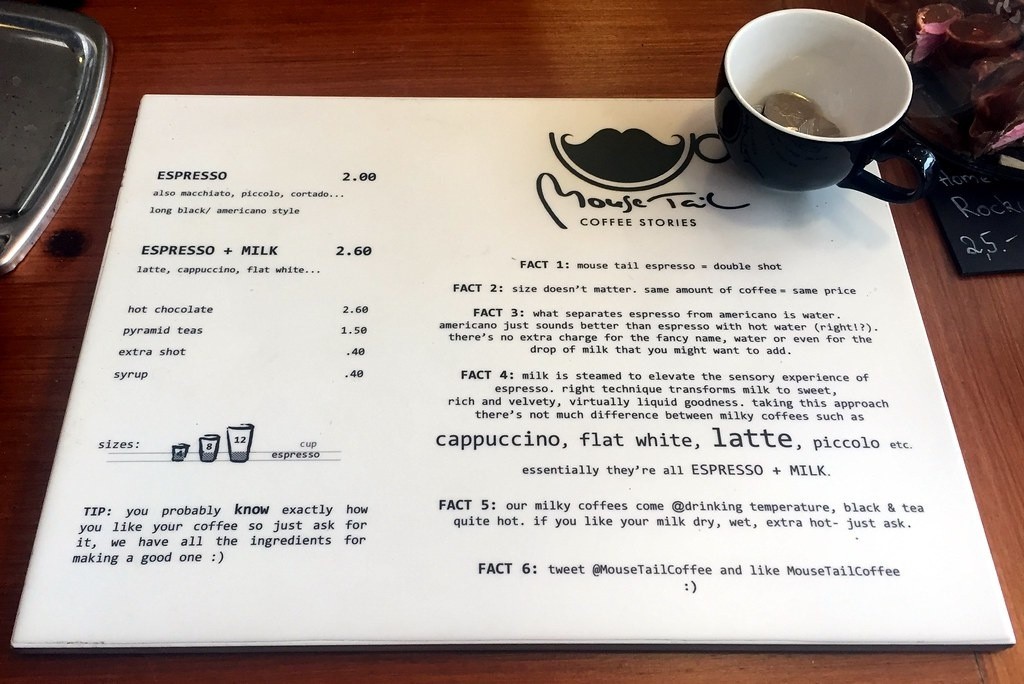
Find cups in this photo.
[716,8,937,204]
